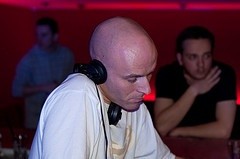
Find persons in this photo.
[155,25,237,138]
[12,15,75,153]
[25,16,184,159]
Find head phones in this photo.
[72,59,123,126]
